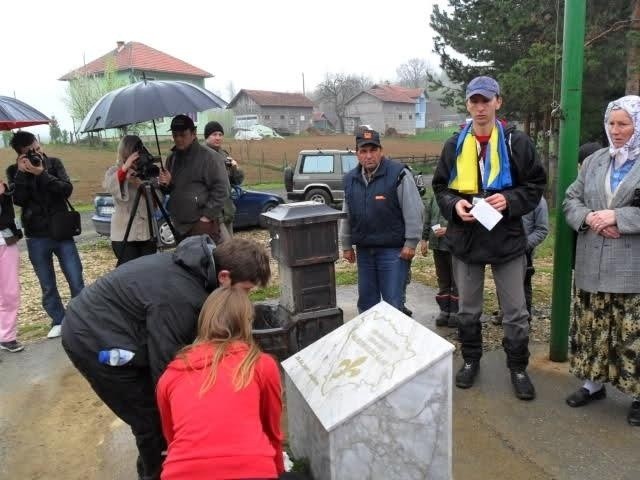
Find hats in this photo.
[204,120,224,139]
[465,76,500,103]
[354,128,381,149]
[167,115,194,134]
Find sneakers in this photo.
[510,368,536,400]
[456,358,480,388]
[0,339,24,354]
[46,323,63,338]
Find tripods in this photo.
[114,183,180,268]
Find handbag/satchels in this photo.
[49,199,82,240]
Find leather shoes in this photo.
[627,394,640,426]
[566,380,607,408]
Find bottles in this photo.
[97,348,134,367]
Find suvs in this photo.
[284,148,426,210]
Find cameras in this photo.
[24,146,42,168]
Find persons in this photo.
[490,195,548,334]
[562,93,640,427]
[421,194,460,327]
[430,76,549,402]
[203,120,245,246]
[154,284,286,479]
[158,113,232,245]
[338,124,426,317]
[0,179,25,354]
[61,233,272,480]
[102,134,166,264]
[5,131,85,339]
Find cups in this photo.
[431,224,441,233]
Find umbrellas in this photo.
[0,95,51,131]
[80,71,229,172]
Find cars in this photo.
[92,177,285,247]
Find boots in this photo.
[447,293,461,328]
[435,293,449,327]
